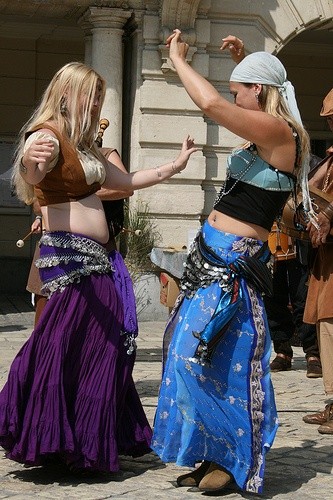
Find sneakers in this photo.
[177,460,236,492]
[270,356,291,372]
[119,452,160,463]
[306,353,323,377]
[302,404,333,434]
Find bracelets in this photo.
[321,204,333,221]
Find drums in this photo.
[268,221,296,262]
[279,187,333,242]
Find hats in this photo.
[319,88,333,116]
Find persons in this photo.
[262,153,322,378]
[302,88,333,434]
[150,29,321,495]
[0,62,203,475]
[26,148,134,330]
